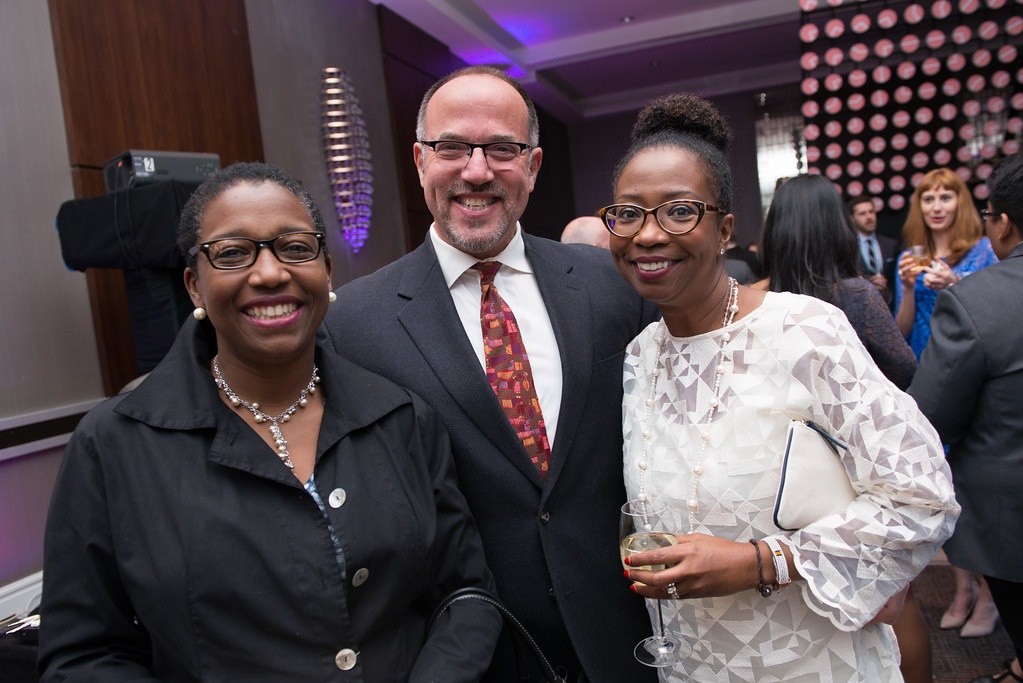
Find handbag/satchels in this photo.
[422,587,575,683]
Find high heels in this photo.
[961,609,999,637]
[940,593,979,629]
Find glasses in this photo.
[979,208,1002,221]
[416,139,536,171]
[600,198,729,239]
[186,231,329,270]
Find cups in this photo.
[909,244,931,267]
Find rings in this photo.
[898,269,904,276]
[660,574,680,599]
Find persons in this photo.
[561,216,611,250]
[599,94,961,683]
[317,66,664,683]
[34,162,503,683]
[721,153,1023,683]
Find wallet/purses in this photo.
[774,418,858,532]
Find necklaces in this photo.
[211,355,319,471]
[634,276,739,534]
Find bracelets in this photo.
[749,536,792,598]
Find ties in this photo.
[469,261,551,482]
[867,240,877,274]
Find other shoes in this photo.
[969,663,1023,683]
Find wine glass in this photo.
[619,498,694,668]
